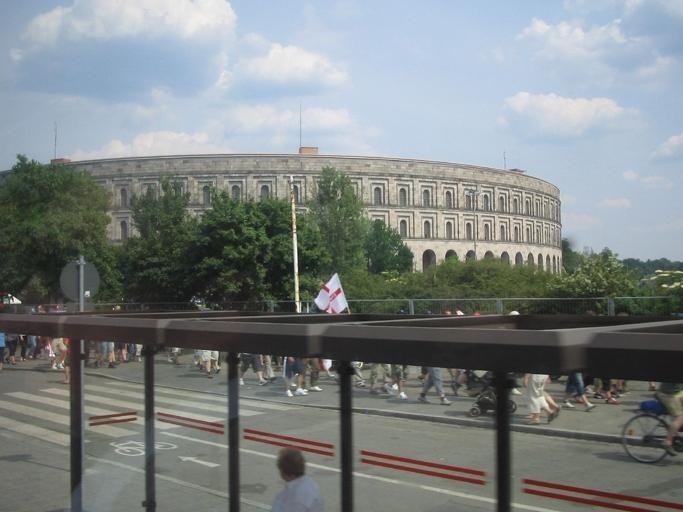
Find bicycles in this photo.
[621,404,682,466]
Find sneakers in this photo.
[286,382,323,397]
[371,383,408,400]
[417,396,431,403]
[562,403,575,408]
[660,442,677,456]
[526,405,560,424]
[258,379,267,385]
[239,378,244,385]
[584,403,596,412]
[440,399,454,405]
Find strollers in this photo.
[464,371,517,418]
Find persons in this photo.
[168,346,221,378]
[271,445,325,512]
[1,331,73,384]
[649,382,682,454]
[237,353,323,396]
[95,341,144,368]
[351,361,493,406]
[508,372,627,423]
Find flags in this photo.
[314,273,347,314]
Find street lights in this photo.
[287,174,314,315]
